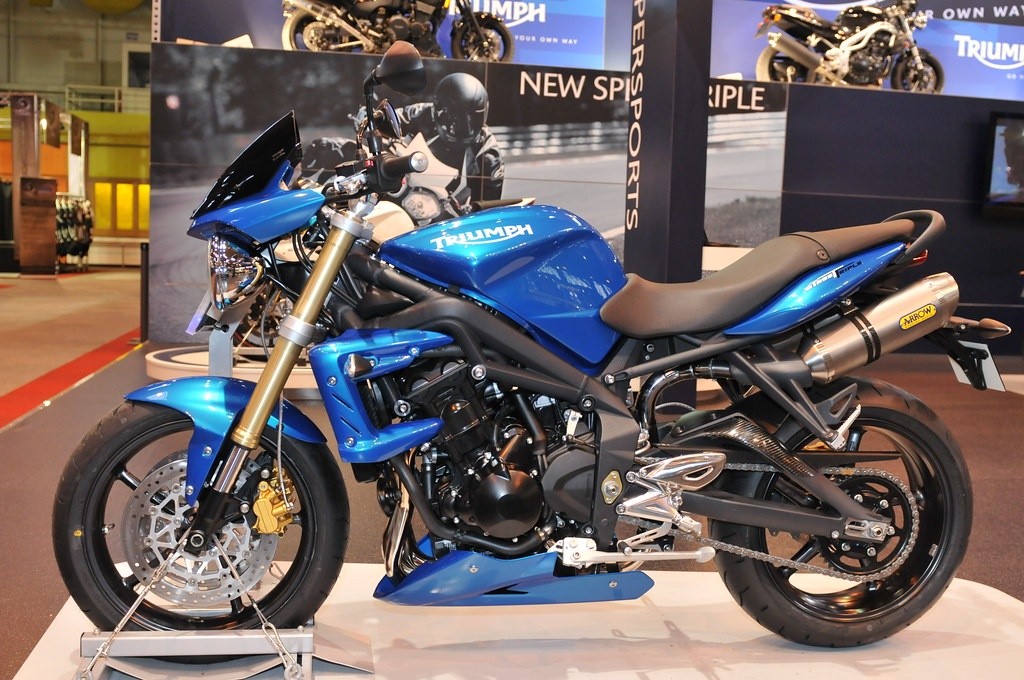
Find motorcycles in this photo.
[213,95,462,364]
[280,0,515,64]
[50,39,1005,666]
[754,0,946,96]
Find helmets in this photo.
[432,71,486,144]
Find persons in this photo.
[305,72,505,211]
